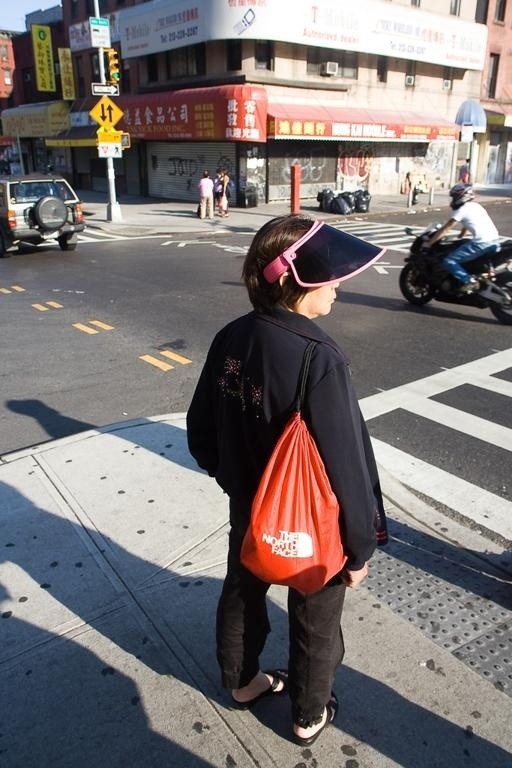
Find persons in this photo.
[193,167,234,220]
[456,158,470,184]
[187,215,390,749]
[418,182,501,295]
[0,162,69,202]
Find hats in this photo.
[263,219,388,289]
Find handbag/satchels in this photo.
[240,416,349,594]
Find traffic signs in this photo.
[89,17,111,49]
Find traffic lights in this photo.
[104,48,121,86]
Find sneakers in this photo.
[457,279,480,293]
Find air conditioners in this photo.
[444,80,452,90]
[406,75,414,86]
[321,61,338,75]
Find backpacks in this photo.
[225,179,233,199]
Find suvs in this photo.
[0,175,85,252]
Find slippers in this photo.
[233,669,290,710]
[292,692,339,747]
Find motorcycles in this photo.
[400,224,512,324]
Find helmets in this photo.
[450,184,475,210]
